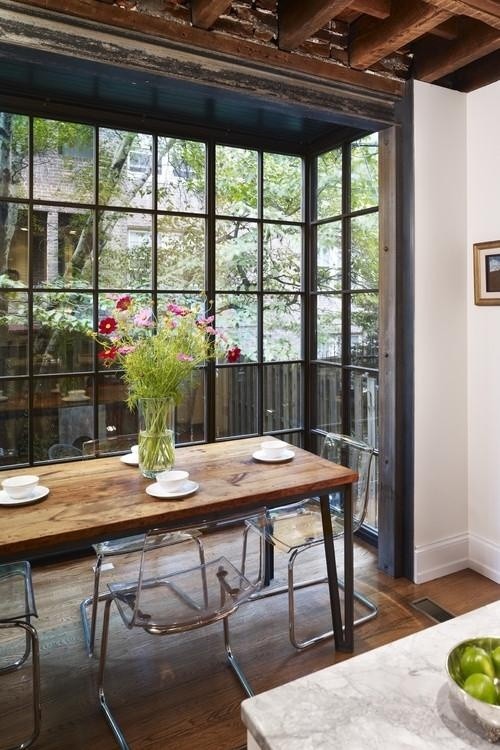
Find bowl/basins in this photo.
[444,635,499,725]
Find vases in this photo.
[137,398,176,478]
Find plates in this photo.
[146,481,201,499]
[119,455,141,466]
[1,485,49,505]
[253,449,295,464]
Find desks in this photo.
[238,599,500,750]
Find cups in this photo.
[155,471,189,491]
[260,441,286,458]
[2,474,38,498]
[130,445,139,460]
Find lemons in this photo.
[459,644,500,704]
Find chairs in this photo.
[78,433,208,656]
[240,432,378,649]
[0,561,42,750]
[79,506,270,750]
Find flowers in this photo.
[83,295,242,469]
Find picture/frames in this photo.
[472,240,500,306]
